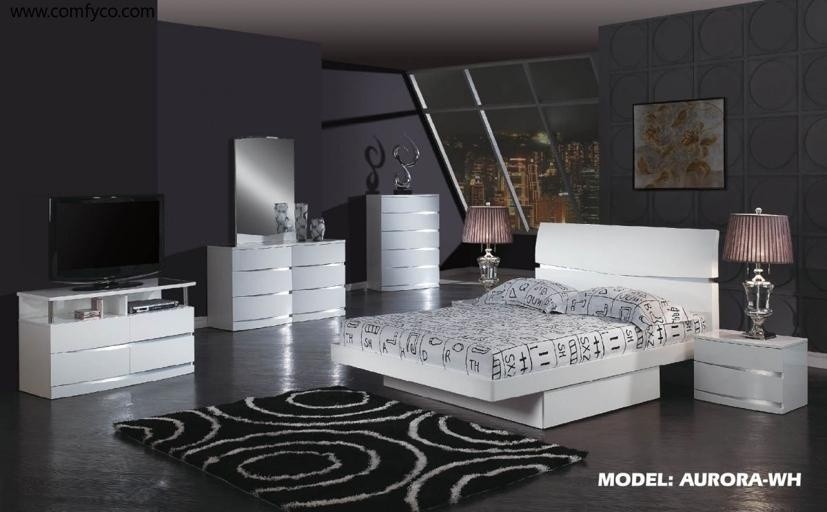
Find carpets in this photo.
[113,386,587,512]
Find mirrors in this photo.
[233,137,297,246]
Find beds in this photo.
[330,223,719,429]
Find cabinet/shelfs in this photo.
[694,329,808,414]
[16,278,196,399]
[367,194,440,293]
[206,239,348,332]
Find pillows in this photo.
[471,277,578,313]
[552,287,690,330]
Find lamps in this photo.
[461,203,513,290]
[721,208,794,338]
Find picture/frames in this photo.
[632,97,727,193]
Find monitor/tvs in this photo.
[48,192,164,291]
